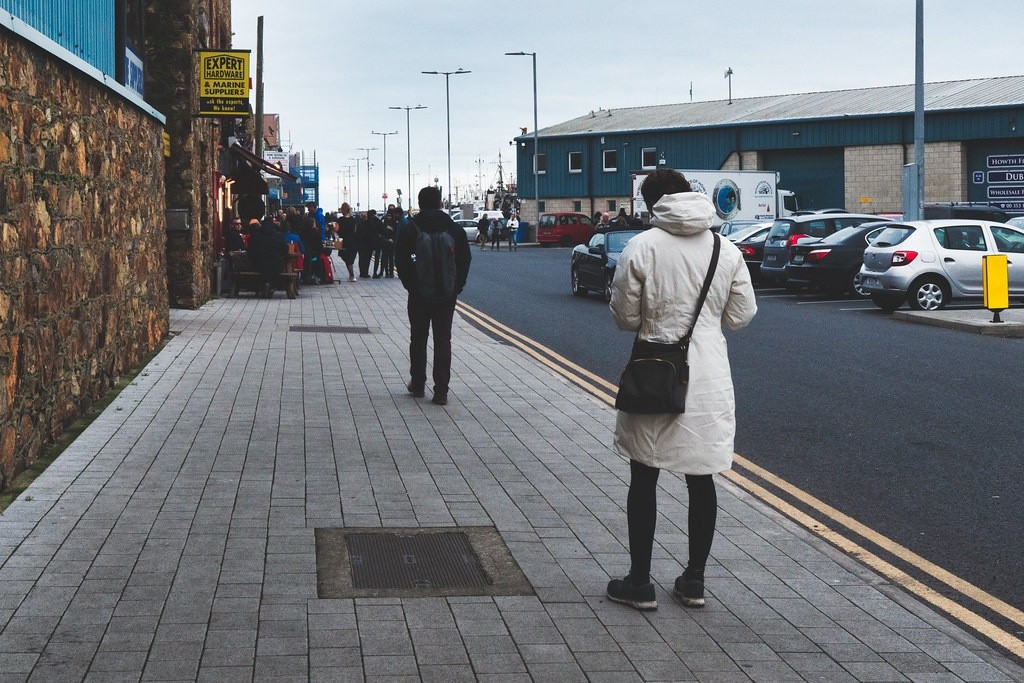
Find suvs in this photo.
[924,202,1010,224]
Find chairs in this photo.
[968,233,983,250]
[948,229,967,247]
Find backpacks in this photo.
[410,218,457,304]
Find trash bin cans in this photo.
[514,222,529,243]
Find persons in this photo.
[478,214,489,249]
[490,217,502,250]
[616,208,629,226]
[593,211,610,229]
[507,214,519,251]
[226,200,326,298]
[607,170,757,609]
[325,202,409,282]
[395,186,472,406]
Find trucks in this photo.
[630,168,800,227]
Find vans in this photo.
[451,210,505,224]
[539,212,595,248]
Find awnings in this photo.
[230,143,298,206]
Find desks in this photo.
[228,250,302,295]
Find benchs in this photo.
[227,272,298,300]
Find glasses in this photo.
[234,223,242,225]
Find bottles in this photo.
[409,253,417,263]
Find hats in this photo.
[250,219,259,225]
[338,203,352,212]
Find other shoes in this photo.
[348,277,352,281]
[376,273,379,278]
[379,272,383,276]
[386,273,389,277]
[351,276,357,282]
[360,274,371,278]
[390,273,394,278]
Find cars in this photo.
[718,219,759,237]
[488,219,510,241]
[982,217,1024,250]
[570,230,647,303]
[785,219,902,300]
[730,208,898,289]
[862,221,1024,313]
[454,220,488,244]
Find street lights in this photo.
[389,105,427,216]
[423,69,473,219]
[371,131,398,214]
[507,57,542,245]
[337,148,380,215]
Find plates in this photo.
[234,251,246,253]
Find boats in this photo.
[439,154,520,210]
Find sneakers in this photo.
[675,576,705,605]
[607,580,658,607]
[432,386,448,405]
[408,379,424,397]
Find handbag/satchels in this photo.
[331,236,343,250]
[615,340,689,415]
